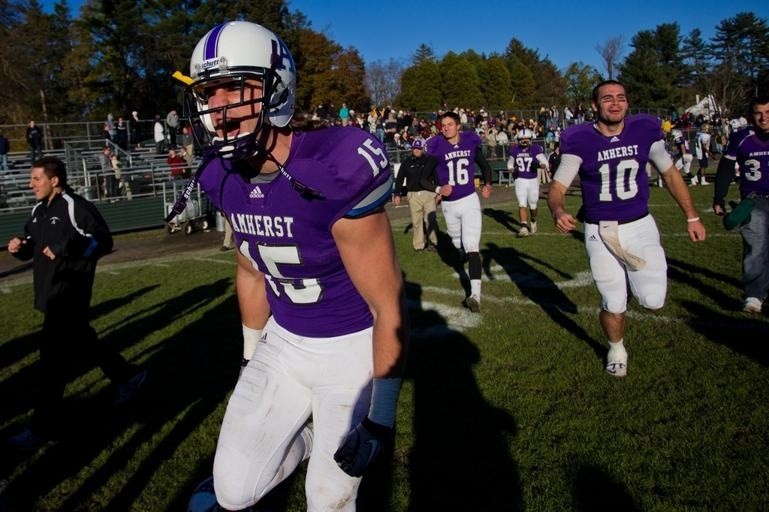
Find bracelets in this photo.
[367,377,403,429]
[687,217,700,222]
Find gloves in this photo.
[440,184,453,197]
[334,415,392,478]
[482,185,491,199]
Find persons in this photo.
[8,157,147,447]
[0,129,10,175]
[713,96,769,313]
[182,21,410,512]
[26,120,44,159]
[547,80,706,376]
[652,109,748,188]
[100,109,234,250]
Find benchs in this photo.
[0,142,203,211]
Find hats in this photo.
[409,138,424,148]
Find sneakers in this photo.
[530,218,538,233]
[743,297,762,313]
[606,339,628,377]
[518,223,530,237]
[466,294,480,313]
[8,426,42,452]
[690,177,709,186]
[115,370,148,403]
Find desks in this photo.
[494,168,513,187]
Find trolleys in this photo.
[158,180,212,233]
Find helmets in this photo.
[518,129,532,146]
[172,20,296,160]
